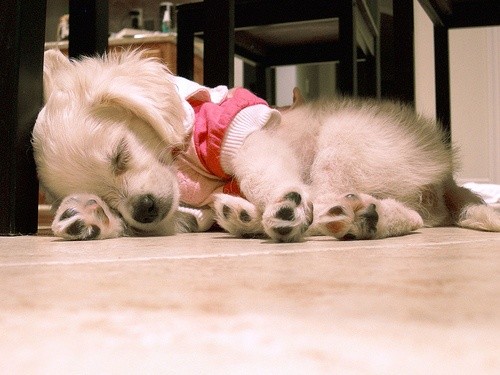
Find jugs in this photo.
[56,14,71,41]
[129,8,144,31]
[159,2,174,33]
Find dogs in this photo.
[29,46,500,244]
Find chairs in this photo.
[175,0,380,101]
